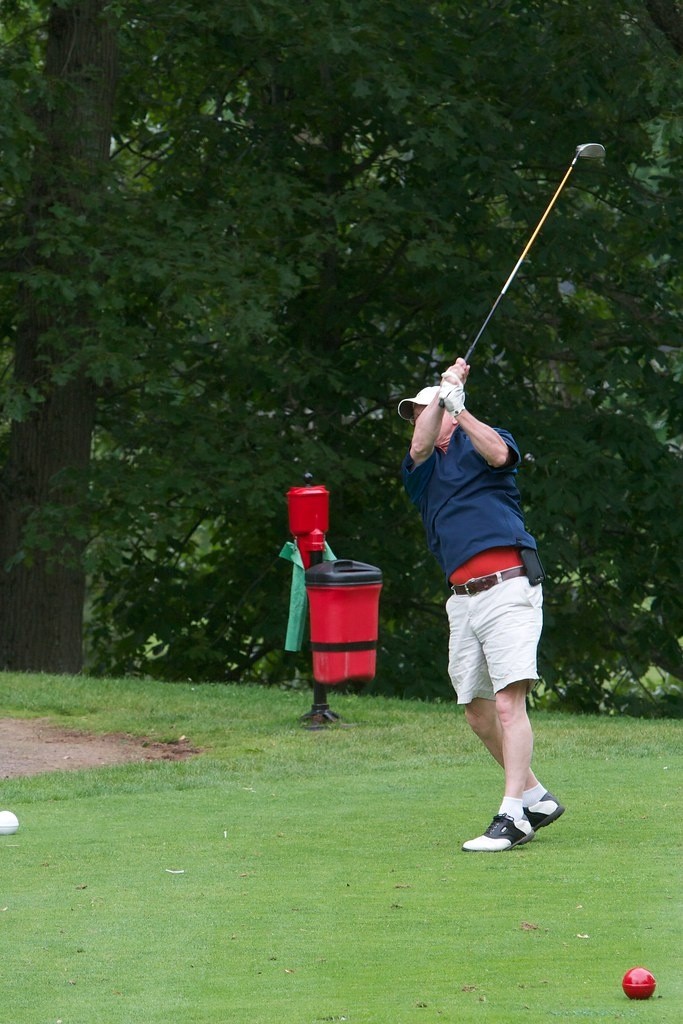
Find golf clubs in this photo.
[438,141,606,410]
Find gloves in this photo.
[438,371,465,417]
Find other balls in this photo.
[621,967,656,999]
[0,811,19,835]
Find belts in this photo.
[453,566,526,596]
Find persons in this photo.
[398,357,566,853]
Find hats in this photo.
[398,385,441,421]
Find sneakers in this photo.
[461,813,536,853]
[523,792,565,832]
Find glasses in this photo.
[409,415,417,425]
